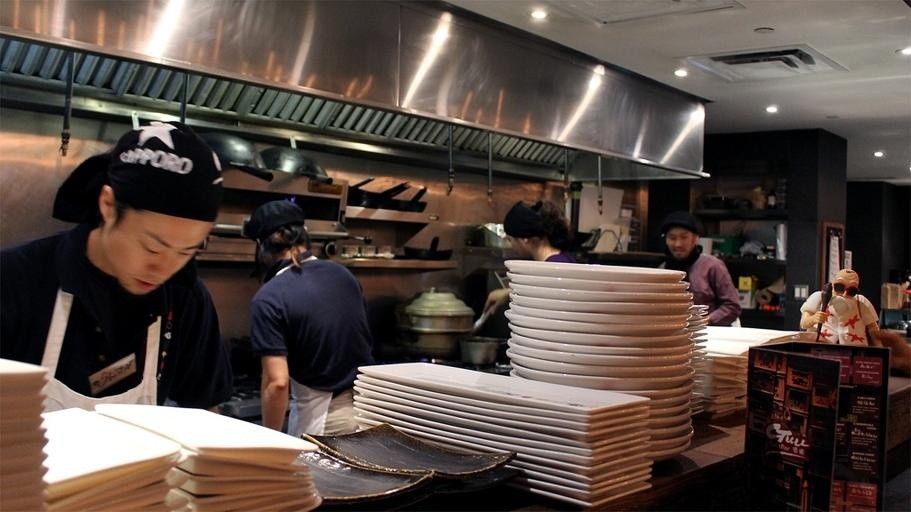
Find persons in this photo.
[878,329,911,372]
[2,122,234,415]
[657,214,742,327]
[482,199,575,316]
[244,200,375,436]
[800,268,883,349]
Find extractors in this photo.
[0,1,710,192]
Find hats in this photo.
[662,211,700,235]
[247,201,303,238]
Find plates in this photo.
[302,419,515,478]
[0,356,328,511]
[308,449,432,504]
[354,356,655,507]
[499,256,712,460]
[706,349,747,418]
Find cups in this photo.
[337,245,395,258]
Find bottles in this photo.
[767,190,776,210]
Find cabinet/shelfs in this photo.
[691,210,787,331]
[193,163,461,271]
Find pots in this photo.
[449,331,499,366]
[405,237,456,260]
[194,125,334,189]
[395,284,477,360]
